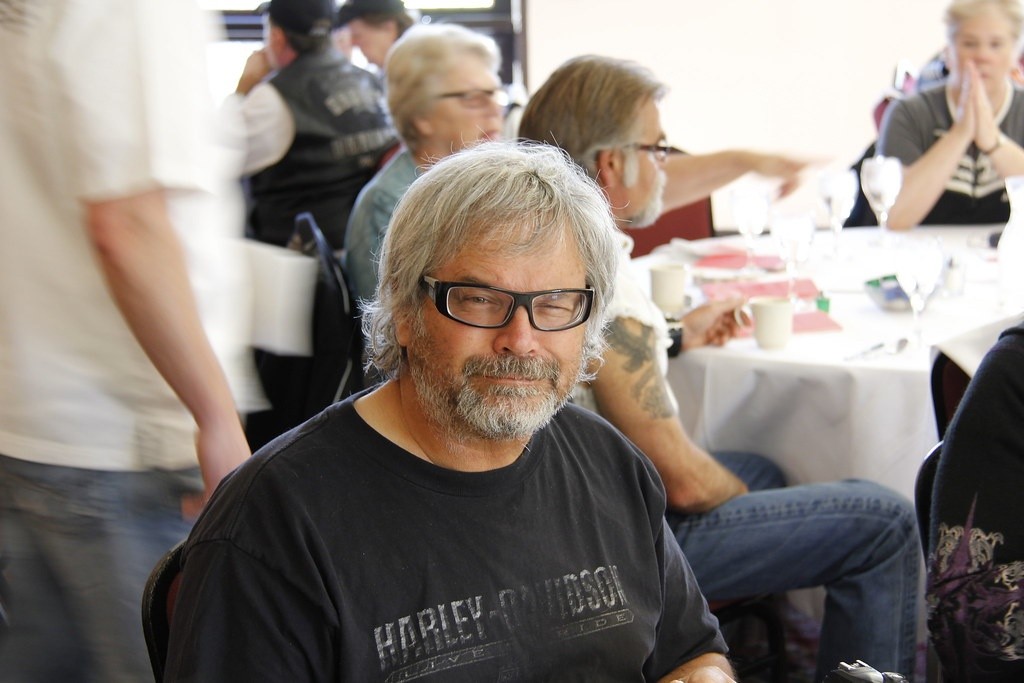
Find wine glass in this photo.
[729,155,902,310]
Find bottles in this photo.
[996,175,1023,309]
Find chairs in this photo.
[141,147,979,683]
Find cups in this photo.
[735,296,794,352]
[649,265,686,312]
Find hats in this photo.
[331,0,403,30]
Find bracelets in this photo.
[978,131,1005,155]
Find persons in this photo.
[855,0,1024,231]
[518,53,918,683]
[926,315,1024,683]
[335,24,831,320]
[233,0,420,409]
[161,141,735,683]
[0,0,271,683]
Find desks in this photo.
[637,219,1024,635]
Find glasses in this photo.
[436,86,511,110]
[419,273,595,332]
[625,133,671,163]
